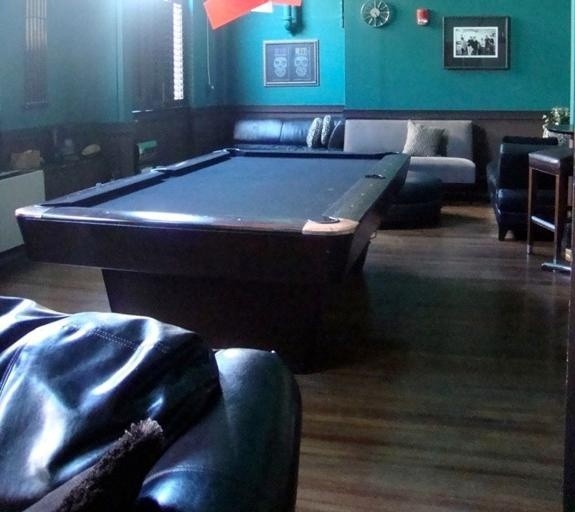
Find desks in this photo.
[546,124,574,135]
[14,147,411,349]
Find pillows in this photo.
[321,115,334,145]
[496,136,557,189]
[328,120,343,149]
[401,121,445,157]
[306,117,322,148]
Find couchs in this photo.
[343,119,476,185]
[232,117,344,149]
[1,349,302,512]
[486,157,556,242]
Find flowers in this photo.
[542,106,569,128]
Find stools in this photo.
[527,146,573,280]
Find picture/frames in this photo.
[441,15,510,70]
[263,40,319,86]
